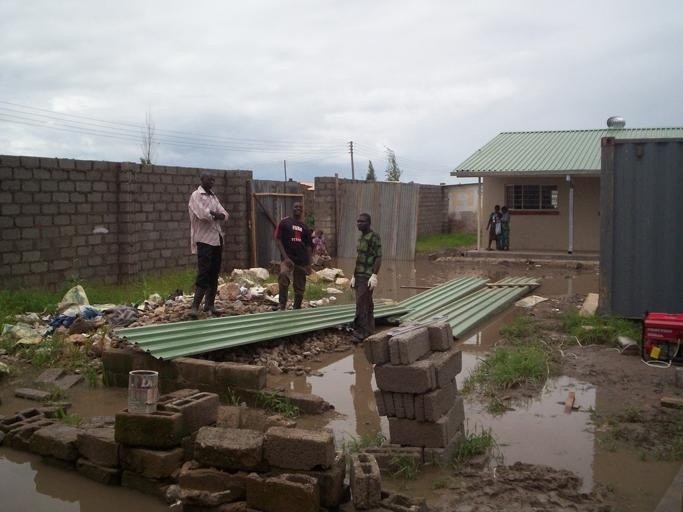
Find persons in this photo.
[348,212,381,345]
[311,231,331,266]
[498,206,511,252]
[186,171,229,317]
[485,204,502,251]
[272,201,314,312]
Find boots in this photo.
[189,286,205,317]
[203,288,219,314]
[293,295,304,309]
[272,287,287,311]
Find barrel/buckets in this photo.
[128,370,159,414]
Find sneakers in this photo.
[349,336,361,343]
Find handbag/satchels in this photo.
[496,223,502,236]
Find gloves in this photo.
[368,273,377,291]
[349,277,355,290]
